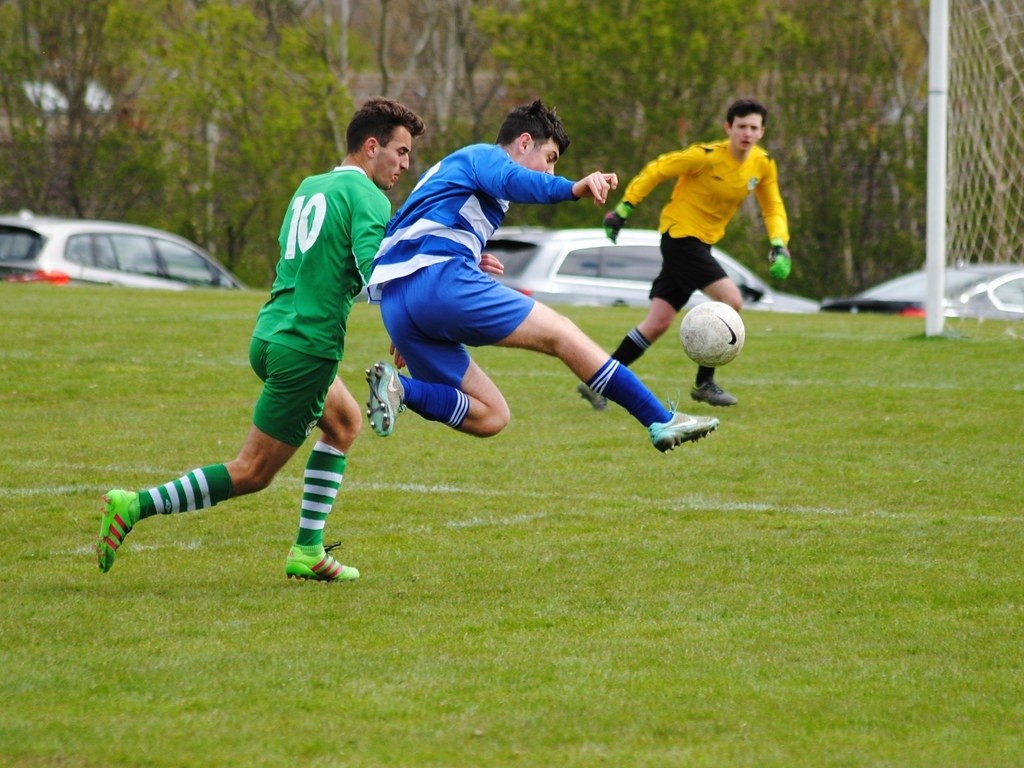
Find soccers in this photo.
[677,300,747,368]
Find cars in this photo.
[817,265,1024,322]
[0,207,249,292]
[475,225,820,315]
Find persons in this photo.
[93,98,504,582]
[576,97,791,411]
[365,99,722,453]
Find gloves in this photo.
[602,202,634,244]
[768,238,792,280]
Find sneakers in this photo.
[690,381,738,407]
[95,488,142,573]
[576,381,609,411]
[365,362,404,437]
[648,392,721,453]
[286,540,360,582]
